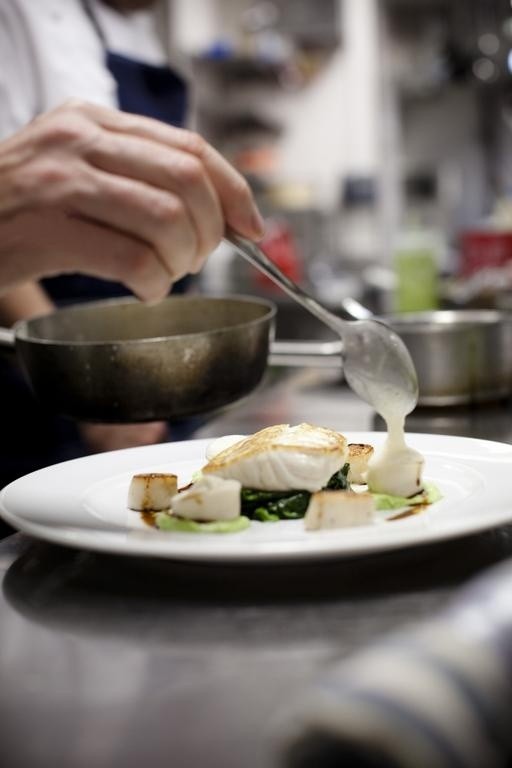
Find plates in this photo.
[0,428,512,569]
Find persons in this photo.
[1,0,195,541]
[1,95,267,304]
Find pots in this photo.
[0,290,281,425]
[267,306,512,408]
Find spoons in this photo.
[220,220,421,419]
